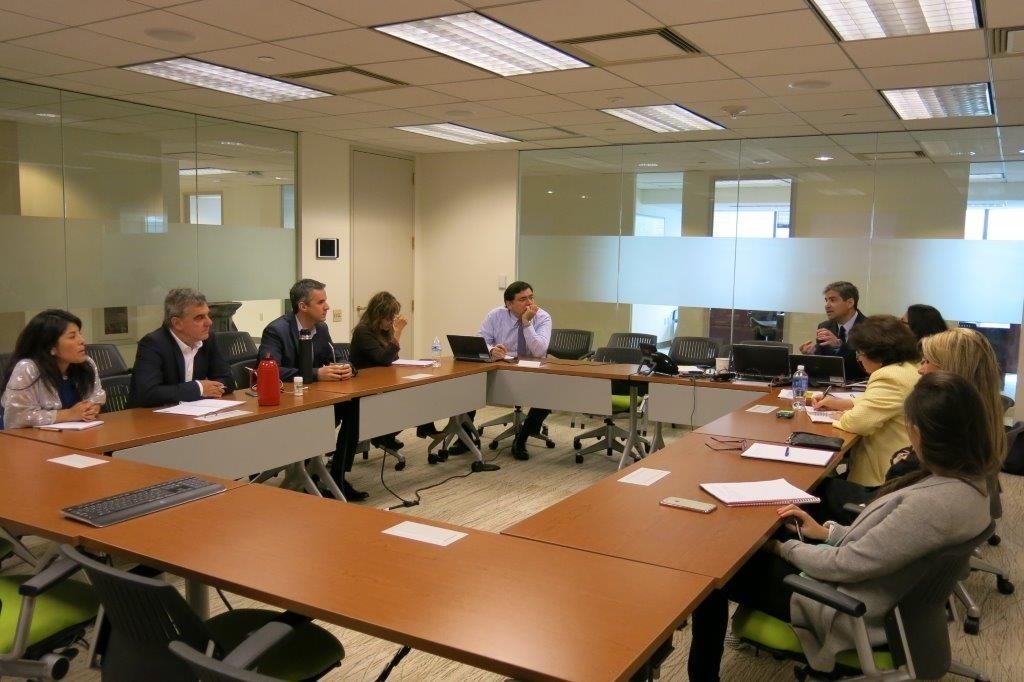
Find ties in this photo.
[517,320,526,355]
[838,326,846,343]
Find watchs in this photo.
[522,320,532,327]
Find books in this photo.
[701,480,820,506]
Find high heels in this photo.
[417,423,443,439]
[371,434,404,449]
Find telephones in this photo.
[637,342,680,377]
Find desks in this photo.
[500,432,844,682]
[1,432,248,547]
[630,368,867,455]
[77,482,714,682]
[283,359,498,503]
[423,355,649,471]
[0,387,351,498]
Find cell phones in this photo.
[776,410,794,417]
[659,497,717,513]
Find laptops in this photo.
[732,344,792,381]
[446,334,502,362]
[789,354,863,389]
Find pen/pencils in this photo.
[794,518,804,542]
[814,386,831,411]
[39,428,62,432]
[785,446,789,456]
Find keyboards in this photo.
[60,477,227,528]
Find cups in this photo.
[716,358,729,371]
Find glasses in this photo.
[856,352,864,361]
[705,437,747,452]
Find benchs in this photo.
[690,390,862,518]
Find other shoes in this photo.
[449,442,468,454]
[303,488,334,499]
[342,480,369,501]
[511,444,529,460]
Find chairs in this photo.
[0,331,406,472]
[805,423,1024,635]
[732,502,998,682]
[0,525,345,682]
[477,328,720,463]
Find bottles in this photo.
[792,365,808,410]
[257,352,280,406]
[431,336,442,368]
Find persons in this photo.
[257,278,370,500]
[0,309,108,558]
[450,280,553,460]
[350,292,445,448]
[125,288,237,408]
[649,281,1006,682]
